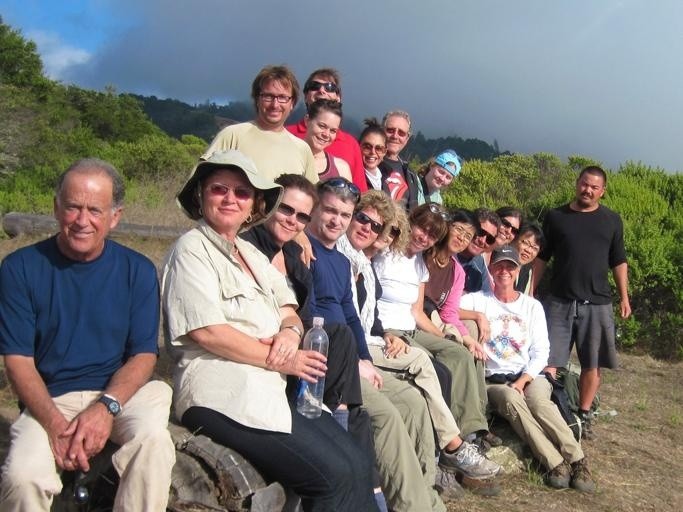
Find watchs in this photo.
[284,326,304,337]
[98,395,121,416]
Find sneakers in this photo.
[438,440,500,480]
[471,437,491,452]
[570,460,595,490]
[481,432,502,446]
[435,457,465,499]
[577,412,593,439]
[548,459,574,488]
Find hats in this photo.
[490,246,521,267]
[434,152,461,177]
[175,150,284,227]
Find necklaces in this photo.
[233,248,239,256]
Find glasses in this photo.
[321,179,361,203]
[306,81,337,93]
[386,128,406,137]
[475,228,496,245]
[428,204,452,222]
[278,202,312,224]
[451,223,473,241]
[211,184,252,200]
[355,212,383,234]
[260,93,293,103]
[501,219,519,236]
[72,470,91,503]
[361,144,388,154]
[522,239,540,252]
[390,226,401,236]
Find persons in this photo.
[302,98,354,184]
[458,247,593,489]
[355,205,464,500]
[424,210,503,455]
[205,67,317,183]
[358,117,391,196]
[483,207,521,291]
[161,150,380,512]
[455,208,502,294]
[380,111,418,219]
[239,173,387,512]
[335,190,498,497]
[294,178,446,512]
[417,153,461,205]
[511,224,546,297]
[371,203,500,495]
[1,157,175,512]
[532,166,631,439]
[286,68,368,193]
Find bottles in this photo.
[295,316,329,420]
